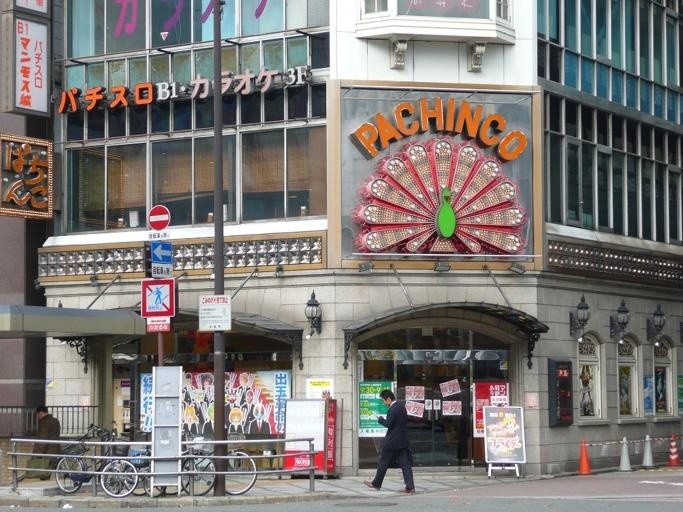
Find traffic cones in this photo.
[615,436,636,472]
[573,437,595,476]
[664,433,683,467]
[639,433,660,470]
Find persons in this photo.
[361,387,417,494]
[27,403,61,478]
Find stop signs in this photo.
[148,204,171,233]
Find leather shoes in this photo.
[364,481,380,490]
[399,487,415,493]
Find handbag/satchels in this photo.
[25,458,52,477]
[390,449,413,466]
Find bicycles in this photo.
[55,423,258,497]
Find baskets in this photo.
[114,437,129,455]
[228,433,246,449]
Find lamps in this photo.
[304,289,322,336]
[569,293,666,342]
